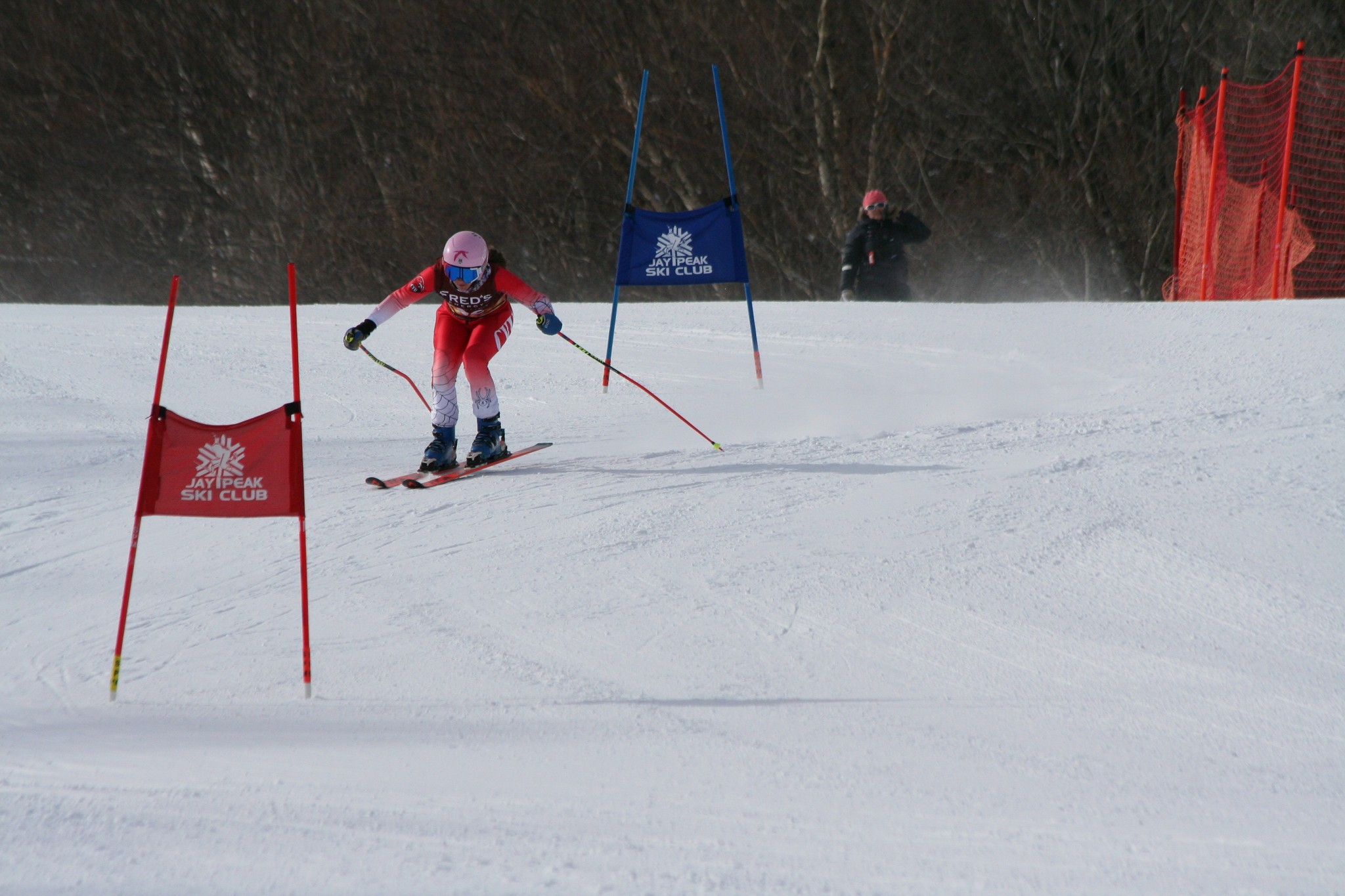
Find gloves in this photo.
[536,314,562,337]
[344,319,377,351]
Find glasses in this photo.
[865,203,886,212]
[444,261,481,284]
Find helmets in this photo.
[442,229,488,268]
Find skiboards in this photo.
[366,443,553,490]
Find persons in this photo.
[343,231,563,469]
[839,190,932,302]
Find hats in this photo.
[863,190,886,208]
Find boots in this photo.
[424,426,457,468]
[472,418,504,457]
